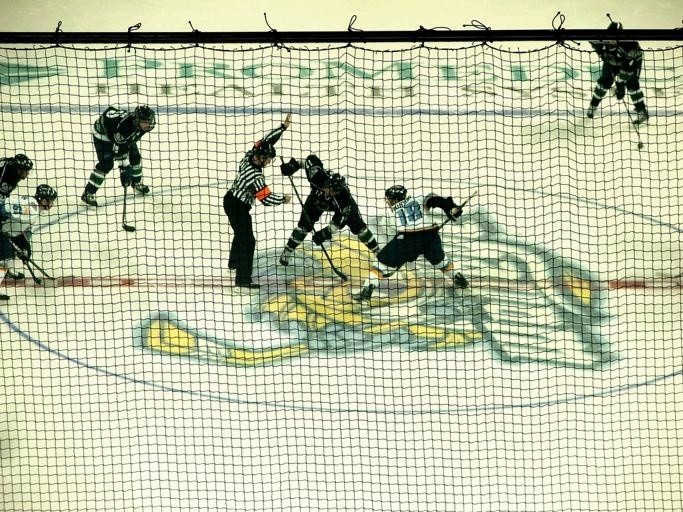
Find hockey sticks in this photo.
[10,238,72,283]
[122,187,134,231]
[280,156,347,281]
[384,192,476,278]
[612,72,644,149]
[25,260,57,287]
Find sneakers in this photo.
[588,105,595,117]
[353,284,376,301]
[452,272,468,289]
[235,278,261,289]
[81,191,98,206]
[279,249,292,266]
[132,182,150,194]
[0,270,25,302]
[631,110,650,125]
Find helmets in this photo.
[251,140,276,164]
[35,184,58,203]
[326,172,347,192]
[385,185,407,202]
[134,106,156,135]
[12,154,33,171]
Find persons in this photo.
[583,20,650,125]
[278,152,382,267]
[0,182,58,301]
[348,183,470,303]
[79,104,158,208]
[1,153,34,200]
[220,110,292,290]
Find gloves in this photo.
[439,196,463,222]
[118,164,133,187]
[616,81,627,99]
[311,226,333,245]
[280,157,300,177]
